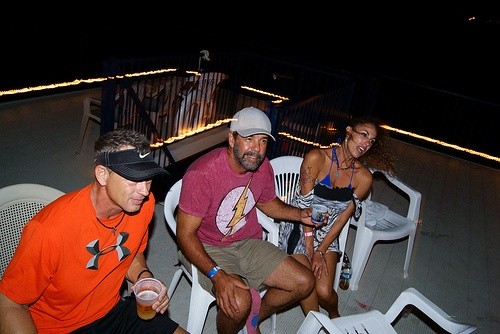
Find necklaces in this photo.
[335,148,354,176]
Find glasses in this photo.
[109,171,153,186]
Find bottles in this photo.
[339,262,352,290]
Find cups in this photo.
[133,277,163,320]
[311,204,328,225]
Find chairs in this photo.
[297,287,478,334]
[0,182,65,289]
[263,156,352,292]
[76,73,228,153]
[167,179,279,334]
[350,167,422,291]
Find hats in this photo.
[96,146,161,178]
[230,106,275,142]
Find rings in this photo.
[316,267,320,269]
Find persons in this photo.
[177,107,330,334]
[278,113,397,319]
[0,127,192,334]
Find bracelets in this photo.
[304,231,315,237]
[208,266,223,280]
[137,270,154,281]
[317,249,324,256]
